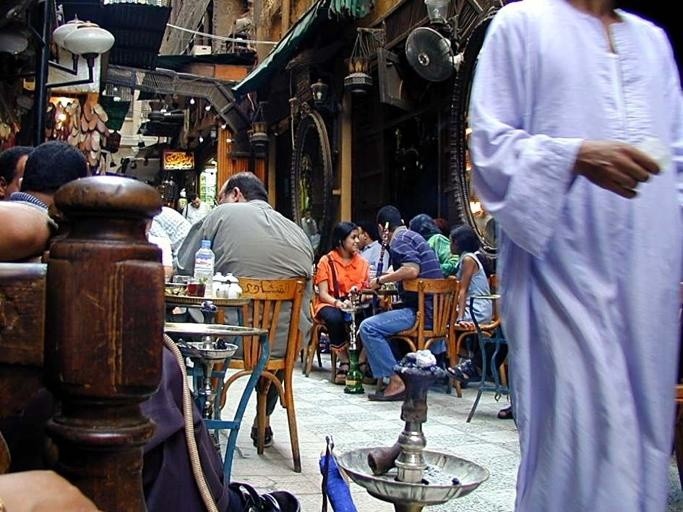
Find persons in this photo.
[467,0,683,512]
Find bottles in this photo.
[193,240,215,297]
[343,346,363,394]
[212,270,240,299]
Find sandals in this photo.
[444,355,492,384]
[497,404,514,420]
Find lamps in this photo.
[344,21,385,96]
[249,98,276,160]
[48,11,84,76]
[45,20,120,90]
[312,80,344,155]
[423,1,460,50]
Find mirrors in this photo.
[289,98,330,254]
[447,6,504,257]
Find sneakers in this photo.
[231,481,301,512]
[251,424,274,449]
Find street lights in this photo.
[1,1,115,150]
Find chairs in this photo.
[209,275,306,472]
[305,269,498,399]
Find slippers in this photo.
[366,386,411,402]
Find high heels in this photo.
[334,359,376,385]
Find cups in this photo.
[170,272,206,298]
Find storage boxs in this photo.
[190,44,211,55]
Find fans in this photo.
[404,27,462,83]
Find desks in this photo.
[164,288,250,323]
[163,322,269,469]
